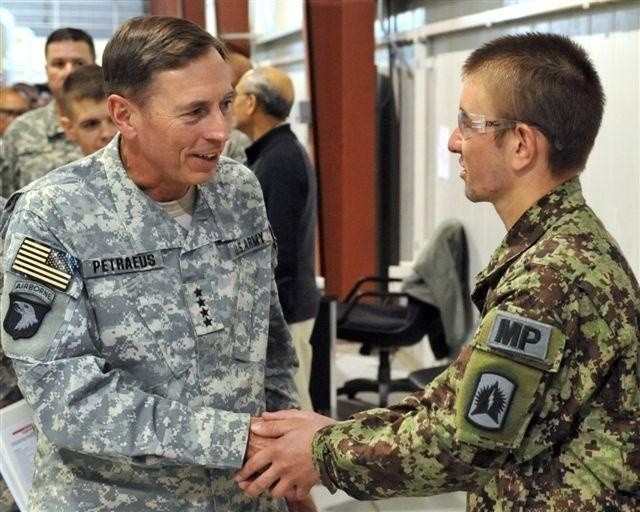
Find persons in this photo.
[0,27,94,197]
[236,33,639,511]
[226,52,254,164]
[60,63,119,155]
[0,83,55,136]
[1,15,316,511]
[230,66,323,408]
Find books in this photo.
[0,397,38,511]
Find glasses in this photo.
[457,110,563,152]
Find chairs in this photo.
[336,218,463,408]
